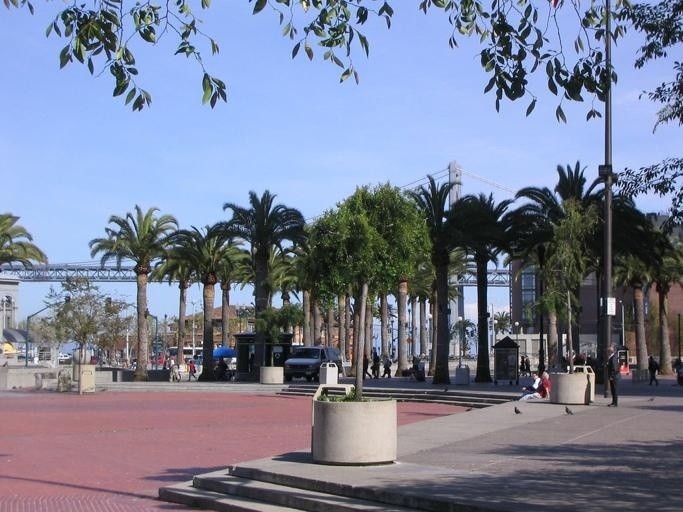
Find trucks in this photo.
[285,346,344,383]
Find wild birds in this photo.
[514,407,520,414]
[464,407,472,411]
[445,386,449,392]
[565,406,573,415]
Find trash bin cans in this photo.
[79,364,96,394]
[320,362,338,384]
[456,364,470,385]
[574,365,595,403]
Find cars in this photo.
[59,354,69,362]
[677,363,683,386]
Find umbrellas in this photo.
[213,346,236,358]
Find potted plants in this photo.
[296,181,439,464]
[251,301,304,385]
[531,197,600,405]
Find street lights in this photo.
[2,296,7,329]
[390,317,394,361]
[617,299,626,346]
[407,308,412,356]
[199,302,204,346]
[514,321,519,343]
[163,313,168,361]
[234,304,246,334]
[191,300,196,359]
[458,316,462,366]
[427,313,433,343]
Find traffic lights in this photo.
[104,297,111,305]
[64,296,70,304]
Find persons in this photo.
[607,346,620,407]
[380,355,392,378]
[648,355,660,386]
[362,353,372,379]
[188,361,197,381]
[216,356,228,370]
[371,352,379,379]
[519,356,551,402]
[407,354,425,382]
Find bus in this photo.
[166,345,203,363]
